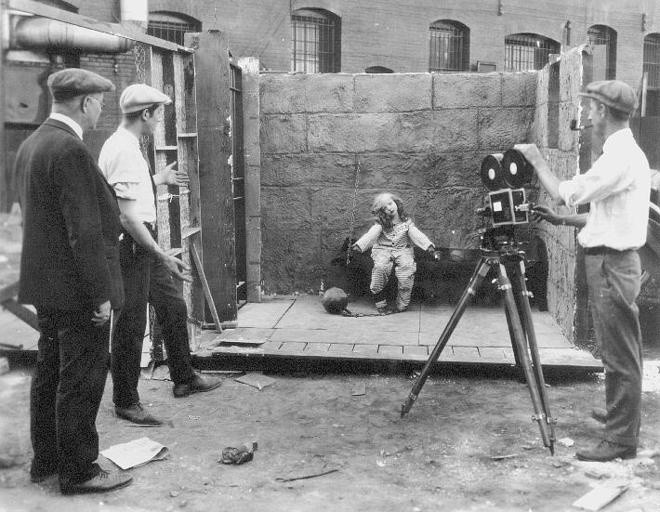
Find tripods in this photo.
[400,226,557,456]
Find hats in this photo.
[578,79,636,113]
[47,67,116,100]
[120,84,173,115]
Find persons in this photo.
[11,66,136,498]
[512,79,651,463]
[351,191,442,313]
[97,81,223,426]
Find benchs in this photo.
[330,246,504,304]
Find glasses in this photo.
[92,97,105,110]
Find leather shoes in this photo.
[174,372,222,398]
[115,402,163,425]
[61,470,133,495]
[592,407,607,423]
[576,440,636,462]
